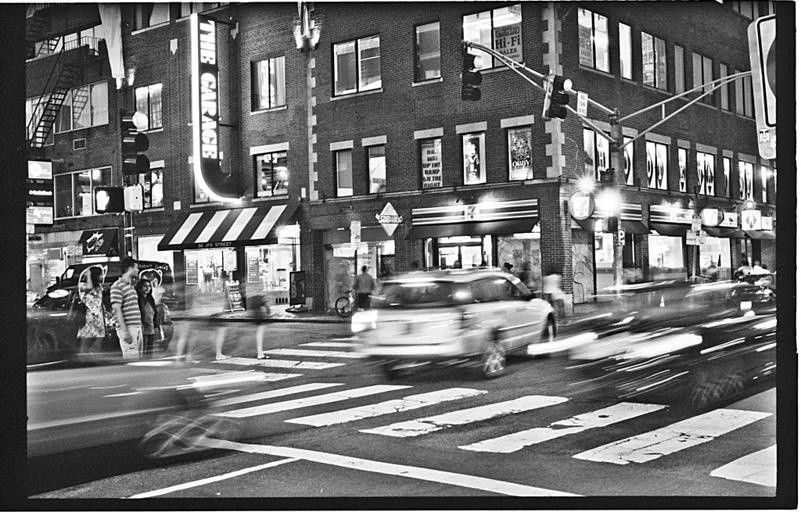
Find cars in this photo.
[725,272,778,307]
[573,277,778,384]
[26,285,173,368]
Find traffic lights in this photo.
[95,186,126,213]
[118,107,152,188]
[123,181,146,214]
[457,50,486,103]
[542,74,573,122]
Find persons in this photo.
[134,278,164,360]
[108,256,143,360]
[149,275,166,345]
[209,292,230,360]
[230,275,276,361]
[172,296,202,366]
[348,256,570,323]
[74,266,107,353]
[610,257,771,300]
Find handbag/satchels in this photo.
[64,291,88,328]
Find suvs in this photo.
[351,267,559,382]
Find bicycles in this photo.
[335,290,360,318]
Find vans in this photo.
[46,259,177,314]
